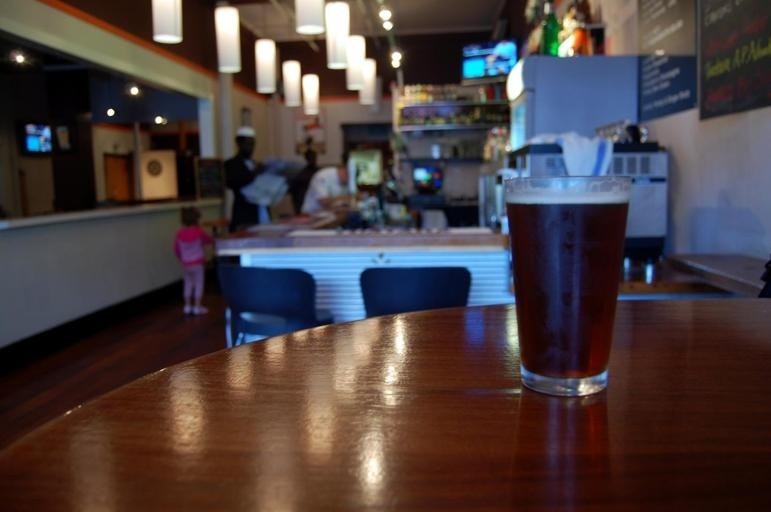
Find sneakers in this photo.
[183,305,209,315]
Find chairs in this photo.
[213,262,334,348]
[358,267,473,317]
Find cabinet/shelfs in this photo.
[395,97,516,164]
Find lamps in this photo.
[147,3,381,118]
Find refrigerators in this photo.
[505,54,641,144]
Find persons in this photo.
[287,148,322,218]
[174,204,215,316]
[300,161,364,220]
[223,127,271,236]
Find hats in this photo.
[236,127,257,138]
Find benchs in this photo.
[664,249,771,296]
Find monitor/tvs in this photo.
[460,35,519,87]
[23,121,55,155]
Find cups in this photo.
[501,176,632,398]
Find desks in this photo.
[0,294,771,512]
